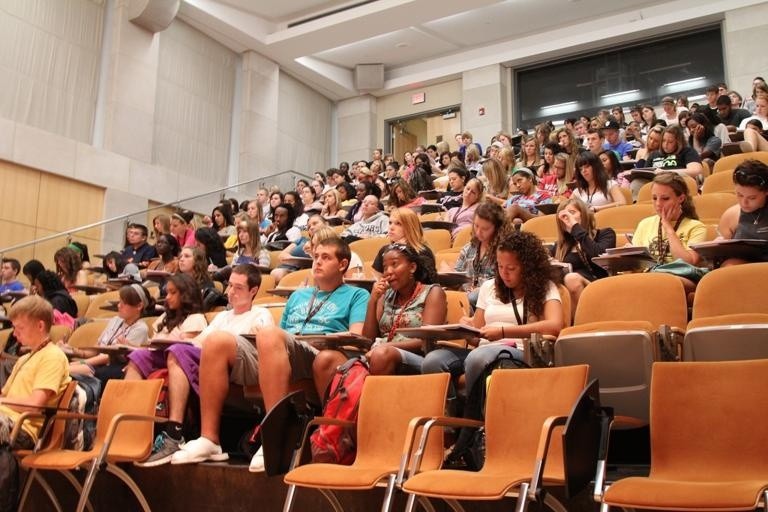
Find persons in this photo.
[0,75,767,490]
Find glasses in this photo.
[736,171,768,187]
[382,245,414,256]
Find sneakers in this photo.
[133,431,186,468]
[170,437,229,465]
[249,445,266,473]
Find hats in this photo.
[600,121,620,129]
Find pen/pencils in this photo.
[715,228,722,237]
[625,233,634,246]
[460,301,468,317]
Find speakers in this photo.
[128,0,184,33]
[353,64,385,90]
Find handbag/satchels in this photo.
[146,370,194,427]
[443,349,535,470]
[64,374,101,449]
[312,356,371,464]
[652,259,709,278]
